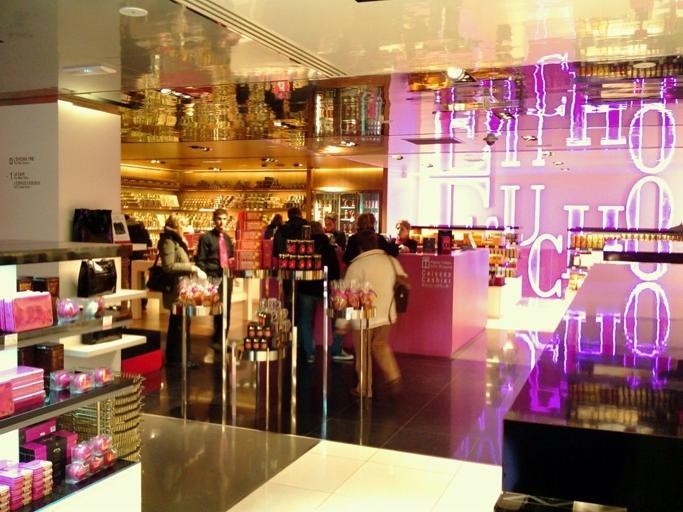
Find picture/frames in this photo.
[499,262,682,510]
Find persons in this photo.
[342,212,400,266]
[194,208,240,367]
[263,213,284,240]
[341,229,410,401]
[294,220,355,366]
[157,212,208,370]
[271,206,310,366]
[389,219,417,254]
[321,212,346,252]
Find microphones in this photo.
[119,326,163,374]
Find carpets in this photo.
[217,232,231,280]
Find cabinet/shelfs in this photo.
[486,232,516,279]
[364,200,377,208]
[575,61,682,78]
[348,224,351,233]
[342,224,346,233]
[569,227,682,249]
[343,210,348,219]
[565,380,683,429]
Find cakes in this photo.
[243,312,272,351]
[278,225,322,270]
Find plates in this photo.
[121,164,179,260]
[560,351,682,435]
[0,238,142,511]
[408,226,518,287]
[565,227,680,270]
[311,190,380,235]
[178,168,312,247]
[63,287,146,361]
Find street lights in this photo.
[146,265,181,292]
[72,207,114,243]
[77,259,118,298]
[393,284,409,313]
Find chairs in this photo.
[306,354,318,365]
[332,350,354,361]
